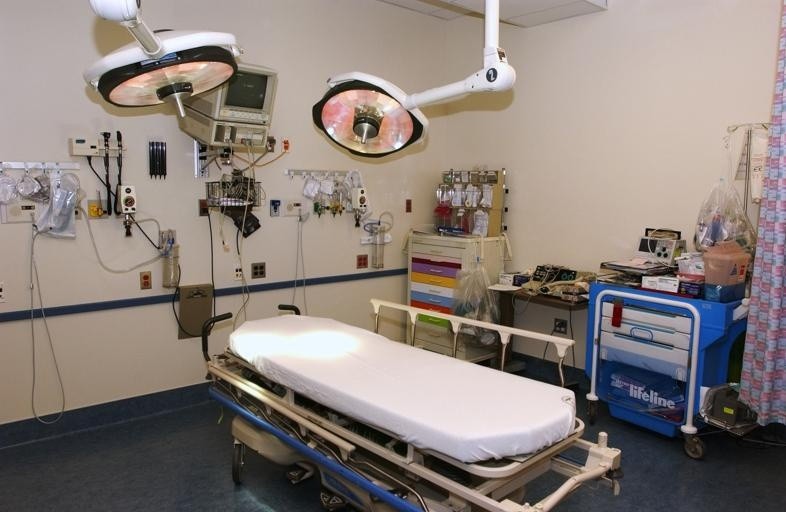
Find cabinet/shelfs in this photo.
[402,227,509,366]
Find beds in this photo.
[186,302,625,510]
